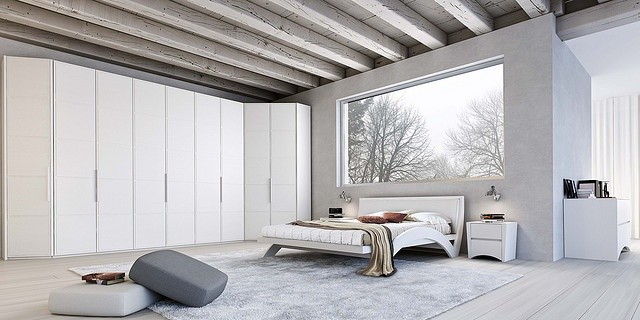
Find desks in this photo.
[562,195,634,263]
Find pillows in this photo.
[381,212,408,223]
[357,215,388,225]
[402,212,451,225]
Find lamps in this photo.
[338,190,352,204]
[484,185,502,201]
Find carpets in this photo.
[69,245,523,319]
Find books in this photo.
[86,280,125,285]
[82,272,125,280]
[481,213,505,219]
[482,219,505,223]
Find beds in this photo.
[259,196,464,279]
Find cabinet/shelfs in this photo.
[53,60,133,258]
[0,56,53,259]
[133,77,194,251]
[195,91,244,246]
[243,103,312,241]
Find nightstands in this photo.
[466,220,518,262]
